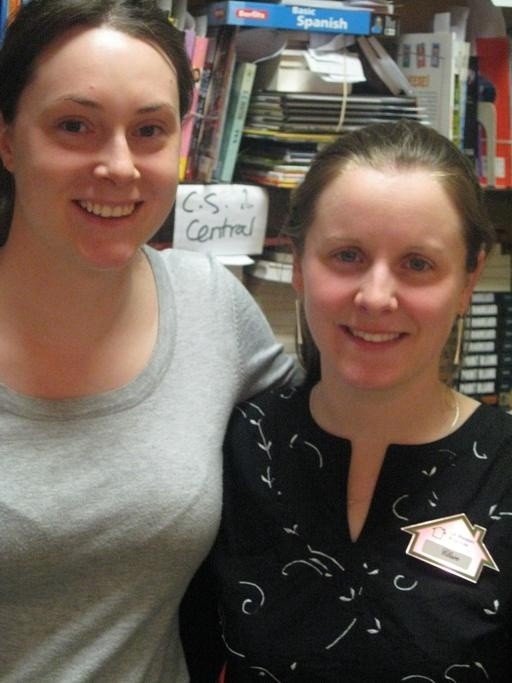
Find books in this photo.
[161,1,511,409]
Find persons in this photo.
[178,117,512,683]
[1,0,297,683]
